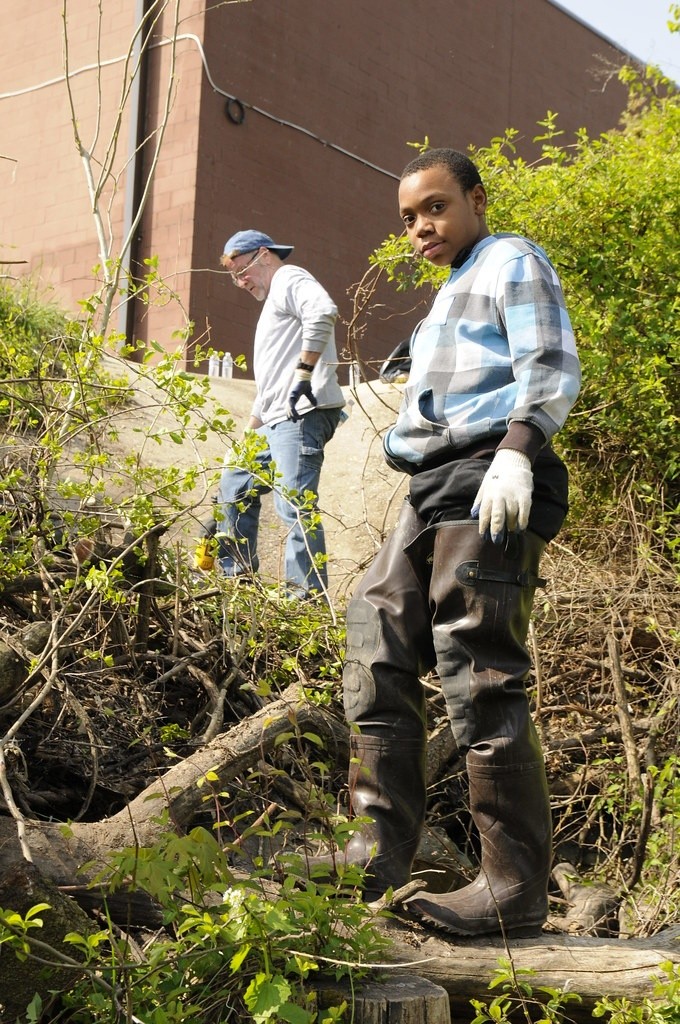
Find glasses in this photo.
[230,251,260,281]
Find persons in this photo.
[271,147,582,939]
[216,228,346,600]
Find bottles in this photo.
[194,496,217,570]
[222,352,233,378]
[349,361,360,386]
[209,351,219,378]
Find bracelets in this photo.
[296,363,314,372]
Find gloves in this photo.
[471,447,535,542]
[286,362,317,422]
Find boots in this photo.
[402,760,554,939]
[280,733,422,899]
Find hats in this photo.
[225,229,294,259]
